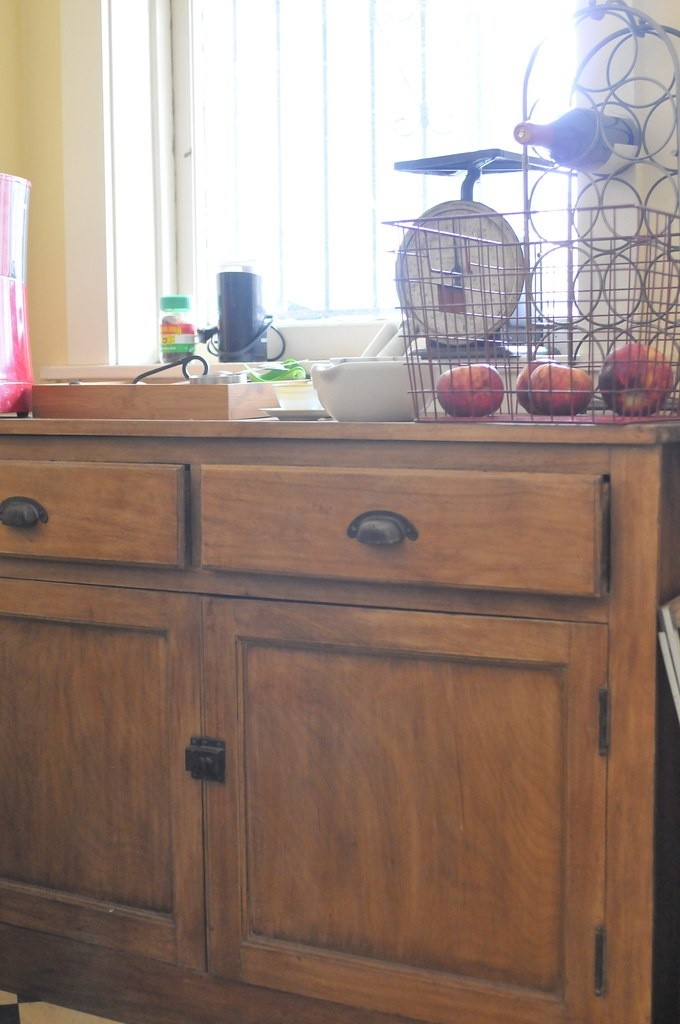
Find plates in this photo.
[258,408,329,422]
[394,200,525,346]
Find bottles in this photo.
[514,108,640,174]
[159,295,196,364]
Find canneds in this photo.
[159,296,195,364]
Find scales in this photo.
[394,148,555,359]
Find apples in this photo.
[514,358,594,416]
[598,342,673,416]
[437,364,505,417]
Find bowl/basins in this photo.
[310,356,440,422]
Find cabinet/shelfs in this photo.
[1,416,680,1023]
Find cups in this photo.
[272,380,315,410]
[189,374,247,383]
